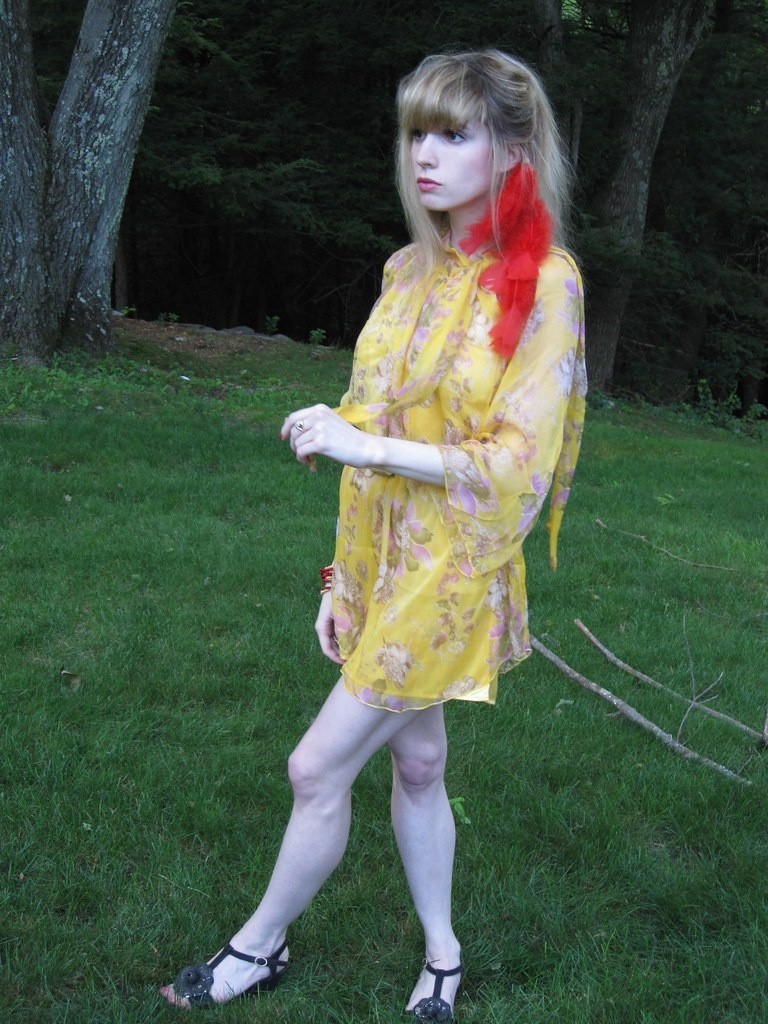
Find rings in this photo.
[295,419,306,432]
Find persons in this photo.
[159,47,589,1024]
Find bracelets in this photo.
[320,566,334,596]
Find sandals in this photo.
[403,954,462,1024]
[158,930,289,1012]
